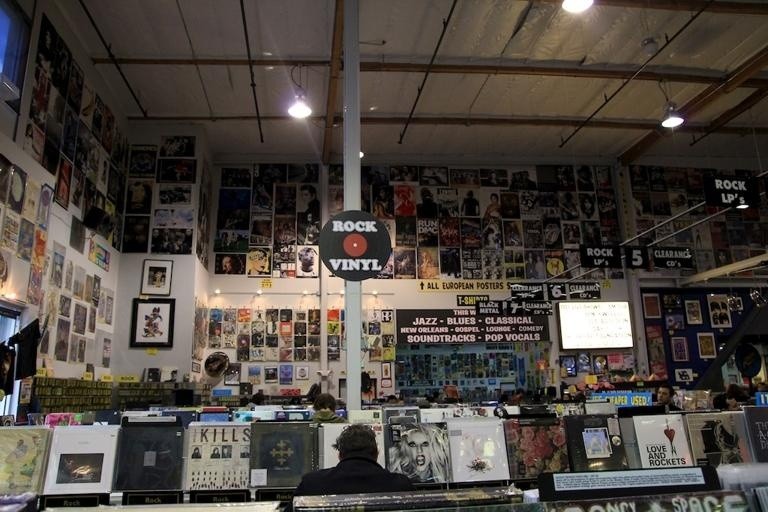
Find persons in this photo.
[23,88,761,394]
[284,424,415,511]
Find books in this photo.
[24,376,763,481]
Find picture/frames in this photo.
[670,336,689,361]
[708,293,732,329]
[140,259,172,297]
[130,297,175,348]
[685,300,703,324]
[697,332,718,359]
[642,292,662,319]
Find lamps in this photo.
[736,187,749,211]
[288,61,312,120]
[644,18,684,129]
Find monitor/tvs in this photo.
[556,297,635,354]
